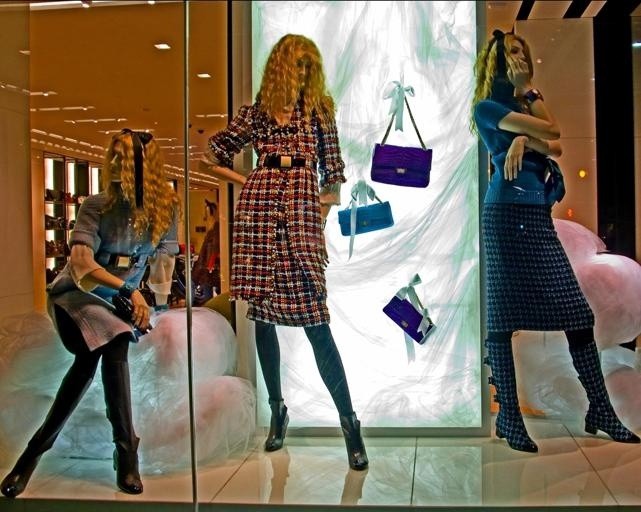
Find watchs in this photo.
[526,89,542,105]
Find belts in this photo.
[109,253,134,270]
[256,155,317,168]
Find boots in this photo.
[569,341,640,442]
[266,399,289,452]
[483,339,538,452]
[340,412,368,470]
[1,375,94,497]
[101,361,142,494]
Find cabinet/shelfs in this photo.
[39,150,180,294]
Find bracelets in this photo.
[320,215,326,230]
[120,280,136,298]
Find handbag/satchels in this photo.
[383,296,436,345]
[371,93,433,188]
[111,294,153,335]
[338,180,394,236]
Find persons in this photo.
[199,200,217,295]
[469,30,641,452]
[0,130,184,499]
[199,33,373,471]
